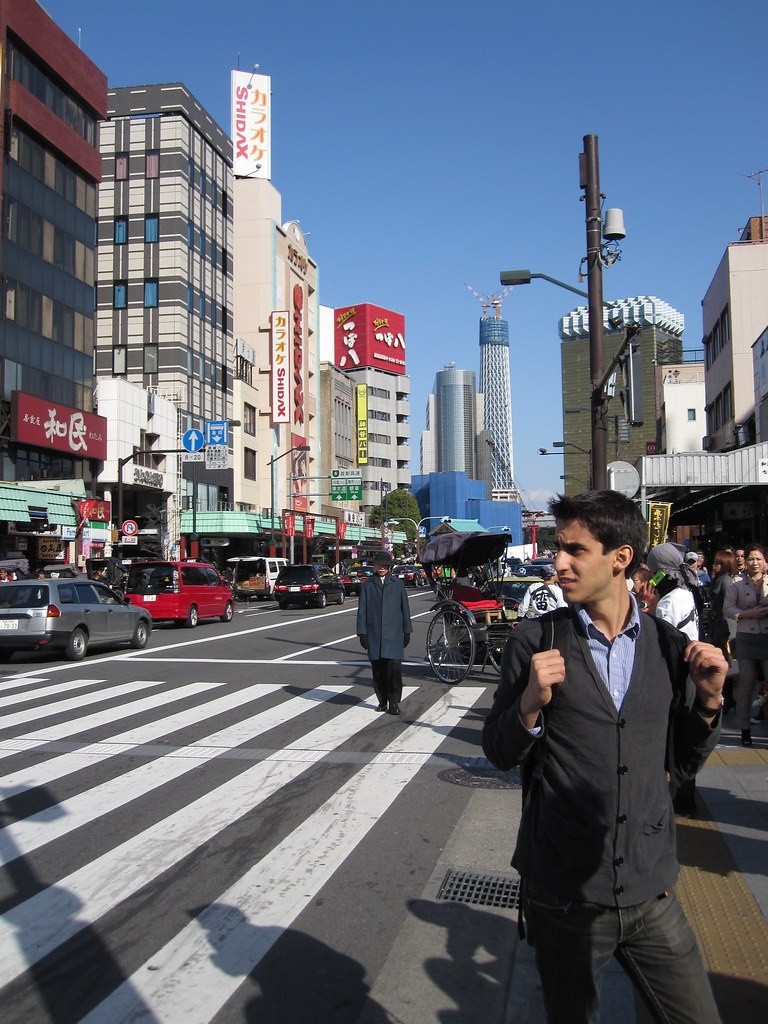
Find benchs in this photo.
[452,583,519,623]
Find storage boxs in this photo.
[238,575,265,589]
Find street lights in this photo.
[559,474,590,493]
[564,407,619,461]
[383,484,413,552]
[553,442,593,493]
[267,445,310,557]
[499,134,617,491]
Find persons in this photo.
[356,551,412,715]
[0,567,13,584]
[636,543,767,747]
[477,489,729,1024]
[509,564,569,629]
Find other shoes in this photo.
[750,704,767,724]
[389,700,401,715]
[375,700,388,712]
[741,729,752,746]
[667,775,697,819]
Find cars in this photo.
[449,554,550,665]
[339,566,375,596]
[0,579,153,662]
[392,566,425,587]
[272,565,346,608]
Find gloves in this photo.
[404,633,411,648]
[360,635,369,650]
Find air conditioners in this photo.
[703,436,710,450]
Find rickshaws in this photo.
[416,530,520,684]
[0,556,78,581]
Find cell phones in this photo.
[648,570,666,589]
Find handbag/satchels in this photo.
[724,640,739,676]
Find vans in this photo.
[224,556,290,600]
[124,561,235,628]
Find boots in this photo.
[723,677,736,715]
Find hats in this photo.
[685,551,698,562]
[372,552,394,566]
[693,549,704,555]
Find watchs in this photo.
[699,693,724,716]
